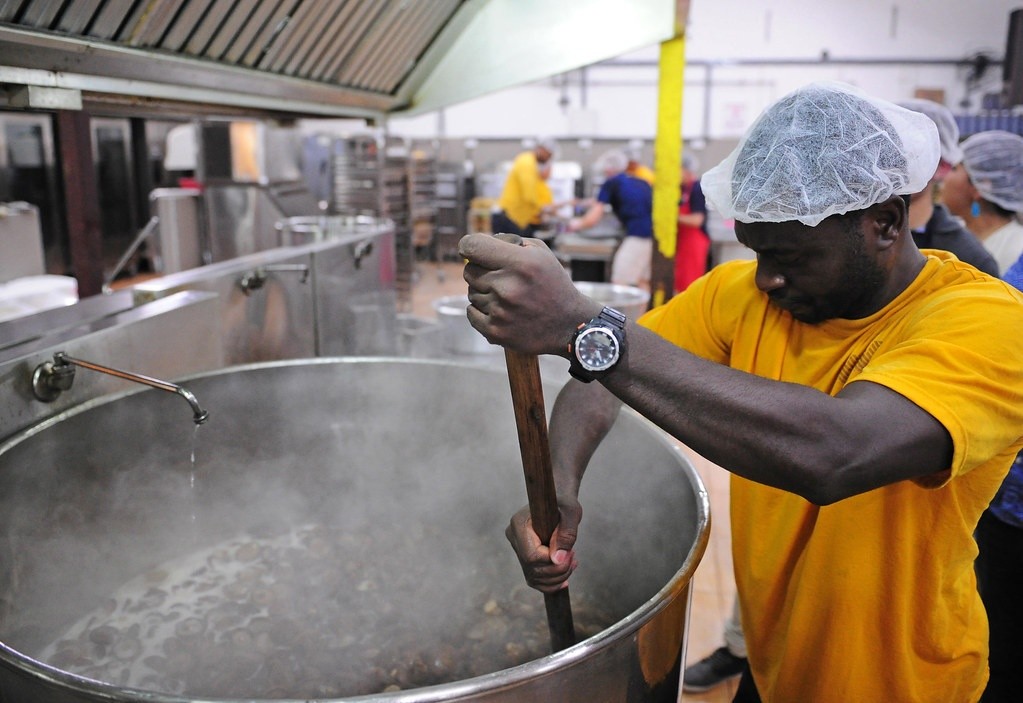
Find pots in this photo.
[1,357,711,700]
[142,188,380,275]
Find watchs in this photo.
[567,305,627,384]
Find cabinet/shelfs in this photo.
[334,124,476,306]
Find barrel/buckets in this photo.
[433,295,493,356]
[573,283,651,322]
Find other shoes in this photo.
[683,647,748,692]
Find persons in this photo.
[457,81,1023,703]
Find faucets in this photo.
[33,351,211,426]
[236,262,309,295]
